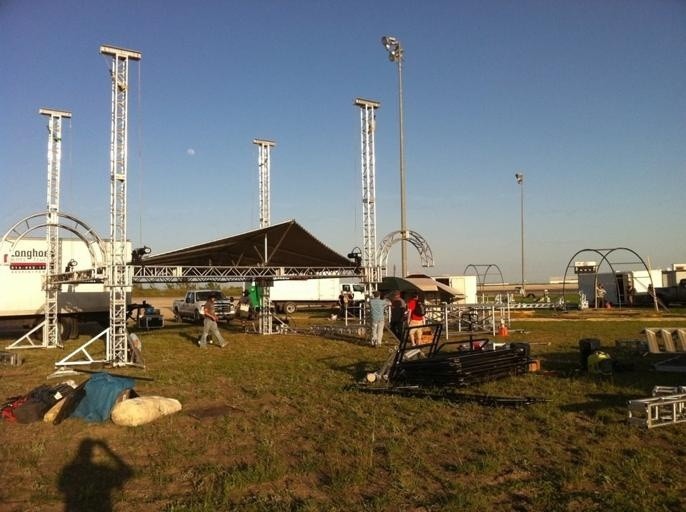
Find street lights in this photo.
[376,33,411,279]
[515,172,526,295]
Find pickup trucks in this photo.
[169,288,238,325]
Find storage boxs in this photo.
[137,313,164,332]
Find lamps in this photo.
[64,257,78,273]
[130,245,152,267]
[346,246,362,267]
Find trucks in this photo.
[0,236,132,344]
[249,269,377,317]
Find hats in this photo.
[207,292,218,299]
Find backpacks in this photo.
[412,299,427,318]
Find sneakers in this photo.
[200,341,230,350]
[370,342,383,349]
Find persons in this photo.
[198,294,229,349]
[369,287,428,349]
[625,278,636,306]
[596,284,607,309]
